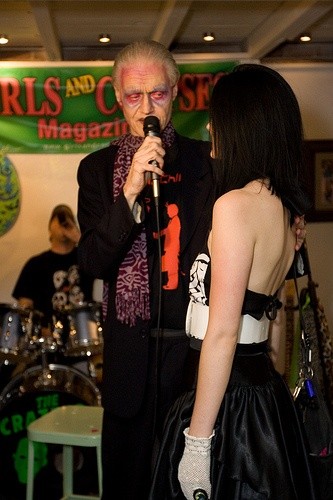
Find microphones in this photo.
[143,115,160,198]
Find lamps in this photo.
[0,35,9,45]
[202,31,215,41]
[98,33,112,43]
[300,31,312,42]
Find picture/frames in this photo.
[299,138,333,224]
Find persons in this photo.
[76,37,306,500]
[141,63,311,500]
[9,204,98,382]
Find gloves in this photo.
[177,427,212,500]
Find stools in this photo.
[26,404,103,500]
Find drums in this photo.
[0,302,44,365]
[55,302,103,357]
[1,364,101,485]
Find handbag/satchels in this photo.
[293,367,332,457]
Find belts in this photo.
[190,336,269,357]
[149,328,186,338]
